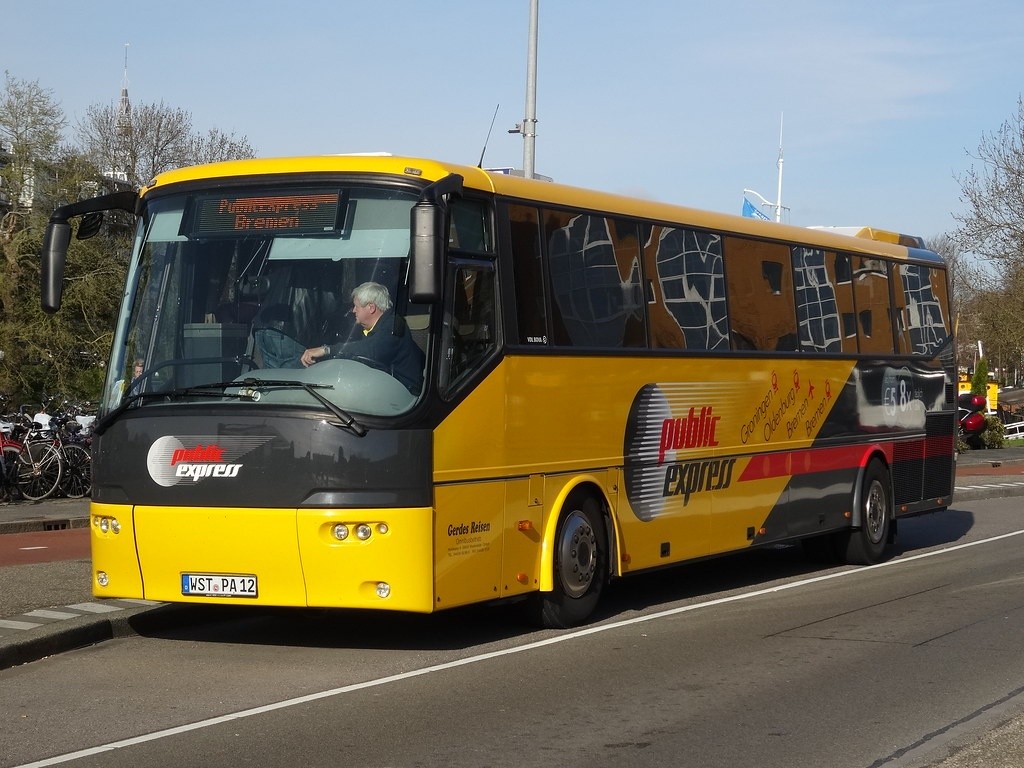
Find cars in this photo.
[957,394,1024,449]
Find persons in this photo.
[300,282,427,397]
[251,259,348,370]
[126,360,153,408]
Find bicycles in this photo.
[0,394,93,504]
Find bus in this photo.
[40,103,964,630]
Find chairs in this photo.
[403,312,452,390]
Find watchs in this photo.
[321,344,329,356]
